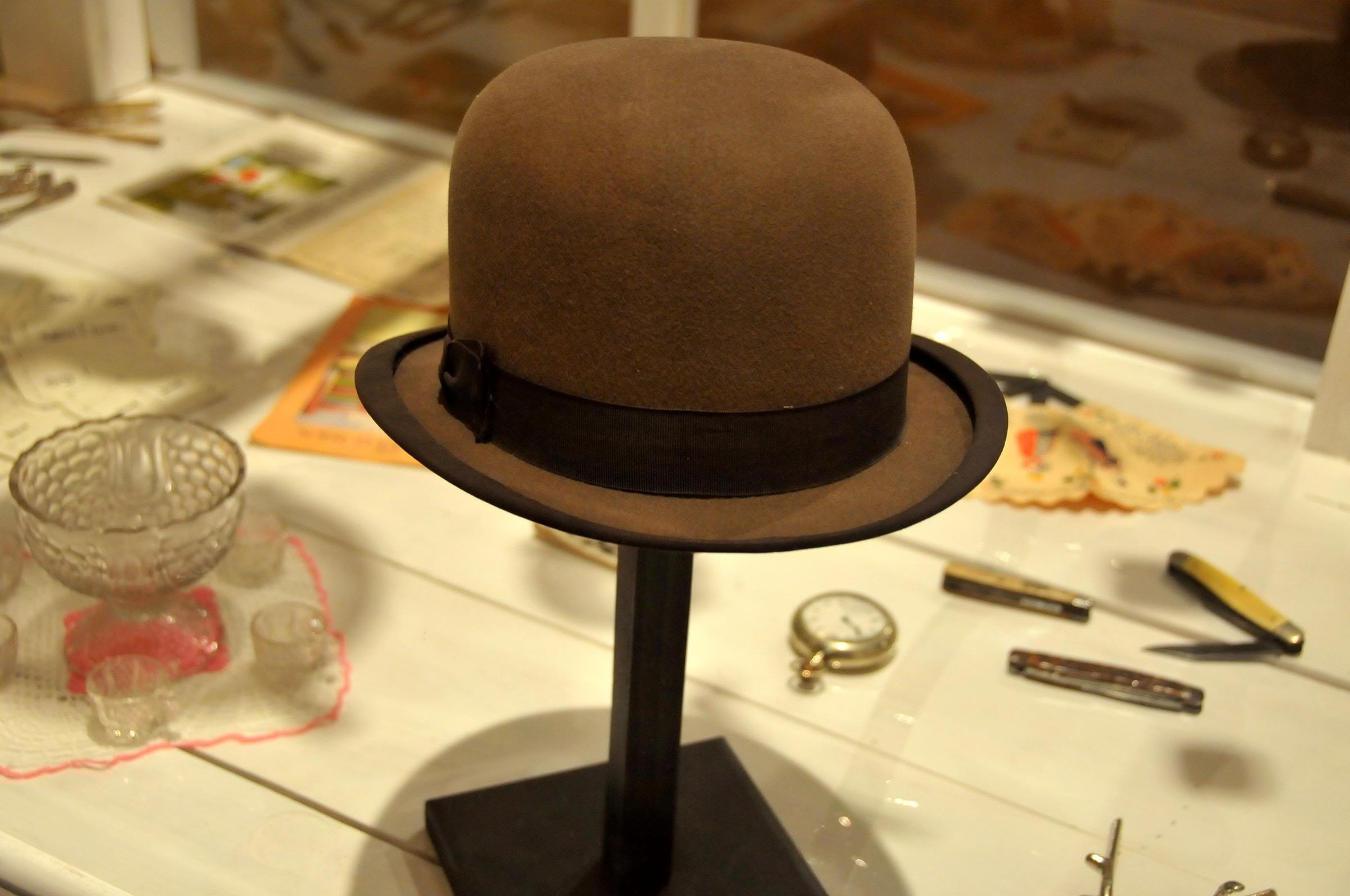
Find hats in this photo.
[353,36,1015,554]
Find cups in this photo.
[0,525,25,690]
[250,601,329,688]
[215,506,288,588]
[85,654,178,747]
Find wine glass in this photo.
[6,413,246,684]
